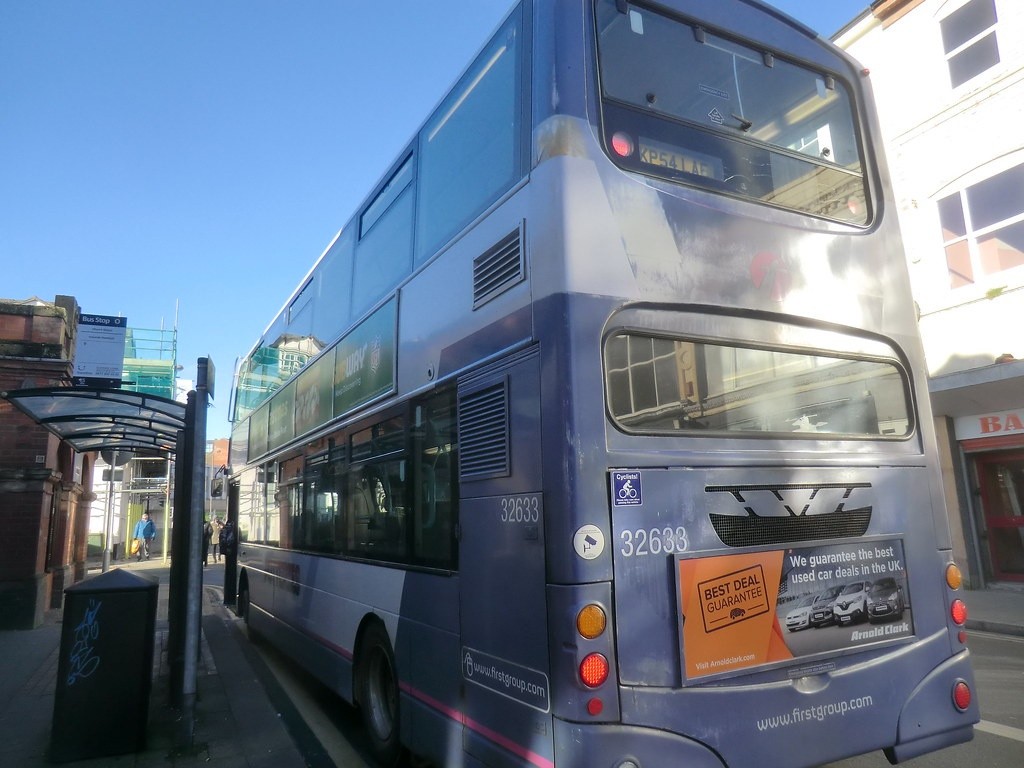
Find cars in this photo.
[786,576,906,632]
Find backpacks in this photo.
[218,517,237,555]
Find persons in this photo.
[133,512,157,561]
[202,513,224,568]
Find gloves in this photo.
[151,537,155,541]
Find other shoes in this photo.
[205,562,207,567]
[215,559,217,563]
[136,556,143,562]
[218,558,221,561]
[145,556,150,560]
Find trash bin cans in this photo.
[48,567,160,763]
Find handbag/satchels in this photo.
[131,538,140,554]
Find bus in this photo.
[210,0,979,768]
